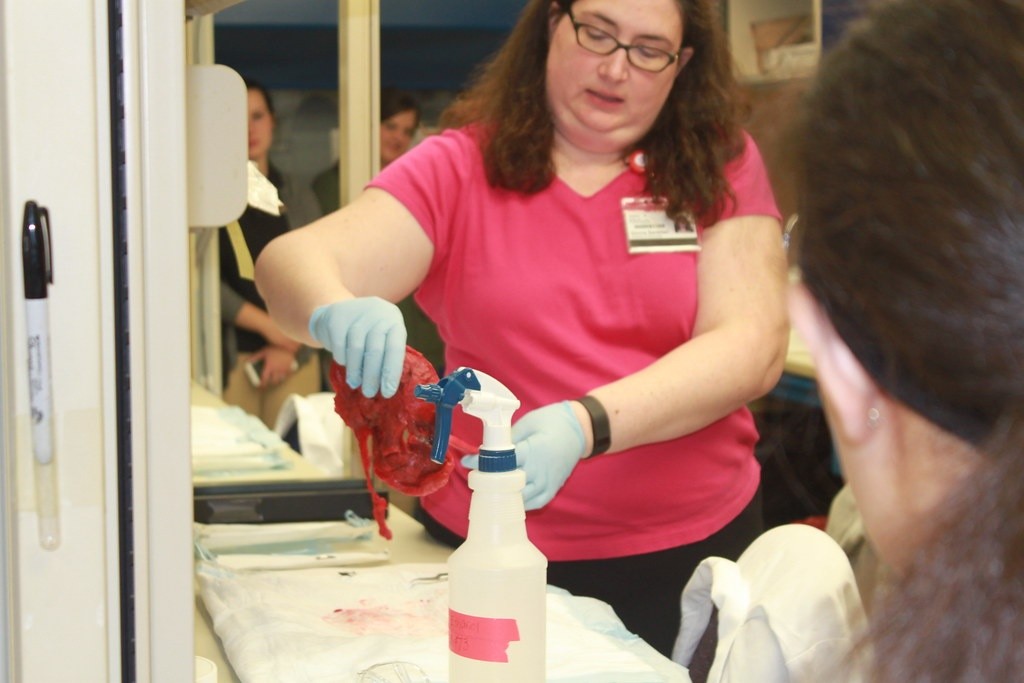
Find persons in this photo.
[309,84,448,387]
[780,0,1023,683]
[217,78,323,437]
[256,2,787,659]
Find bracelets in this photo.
[576,394,613,458]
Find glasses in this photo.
[565,3,682,73]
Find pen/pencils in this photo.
[22,201,60,552]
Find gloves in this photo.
[310,297,407,399]
[460,400,586,511]
